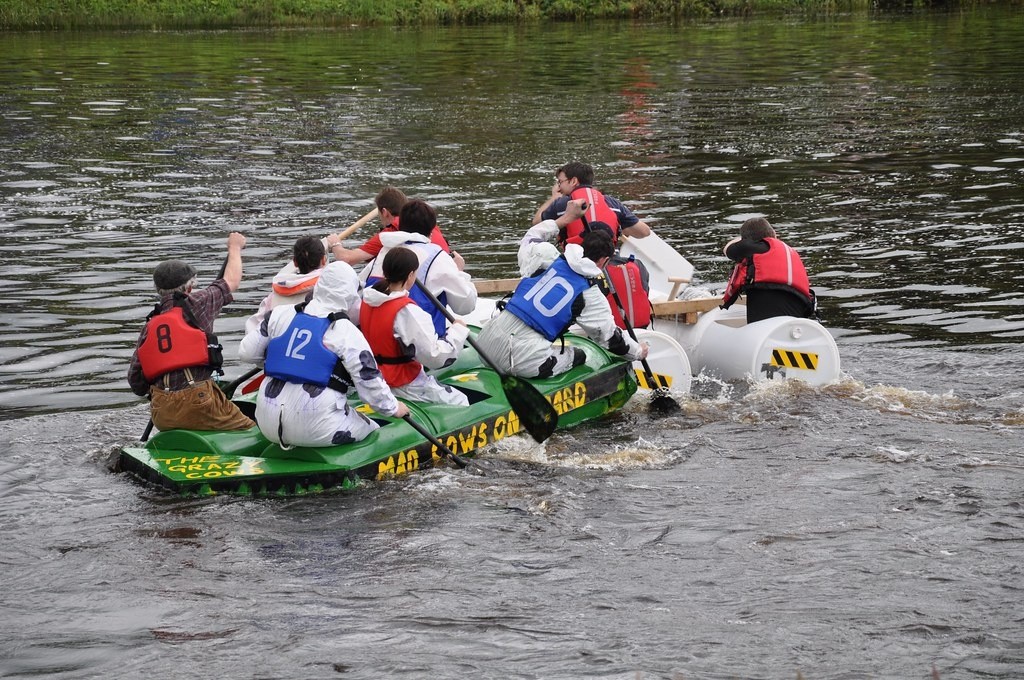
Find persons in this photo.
[238,260,410,452]
[126,231,258,432]
[475,198,650,381]
[244,235,329,372]
[719,217,817,325]
[345,247,470,407]
[326,185,451,267]
[355,197,478,340]
[531,161,651,254]
[578,220,651,331]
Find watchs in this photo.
[327,241,342,253]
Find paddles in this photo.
[141,252,231,441]
[618,229,695,301]
[580,204,682,424]
[276,208,379,278]
[403,414,468,468]
[414,278,560,444]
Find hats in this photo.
[565,222,615,245]
[153,260,197,290]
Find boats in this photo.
[436,283,843,416]
[114,323,646,501]
